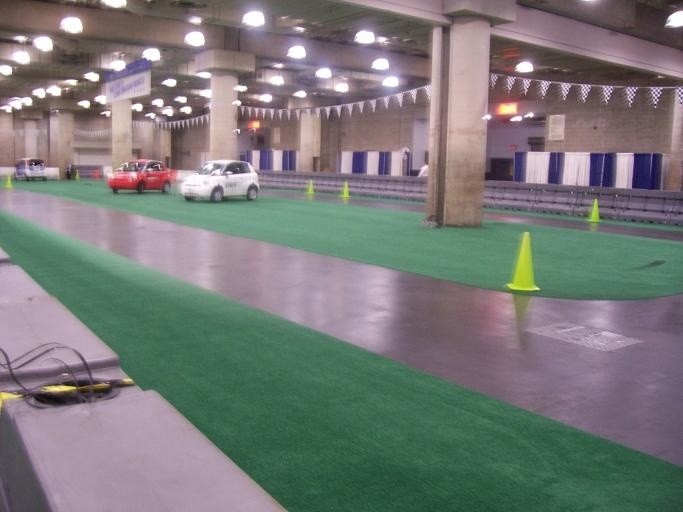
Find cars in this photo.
[108,159,176,194]
[13,158,47,183]
[179,159,260,203]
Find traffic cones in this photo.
[340,182,350,198]
[506,232,541,293]
[94,168,100,179]
[4,175,13,189]
[305,180,315,194]
[74,170,80,181]
[587,199,602,223]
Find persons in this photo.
[416,159,428,177]
[65,160,74,180]
